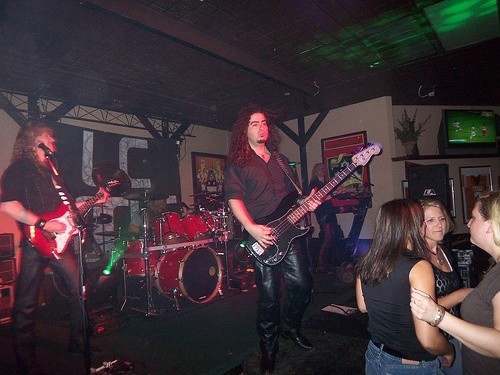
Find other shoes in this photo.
[15,359,45,375]
[67,342,104,357]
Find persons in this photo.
[224,102,330,375]
[307,162,350,276]
[481,124,488,136]
[469,124,477,140]
[0,120,111,375]
[455,120,463,132]
[355,199,455,375]
[416,198,475,375]
[411,192,500,375]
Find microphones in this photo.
[37,140,53,156]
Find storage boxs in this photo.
[458,250,473,264]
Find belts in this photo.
[373,341,413,360]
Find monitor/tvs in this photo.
[436,108,499,155]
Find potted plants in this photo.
[393,107,432,156]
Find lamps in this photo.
[86,265,116,307]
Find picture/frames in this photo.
[402,177,456,218]
[321,130,372,213]
[459,164,493,224]
[192,151,227,205]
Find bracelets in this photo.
[428,304,445,327]
[36,218,47,228]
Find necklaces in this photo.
[425,244,444,265]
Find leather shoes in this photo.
[280,329,315,352]
[259,353,276,375]
